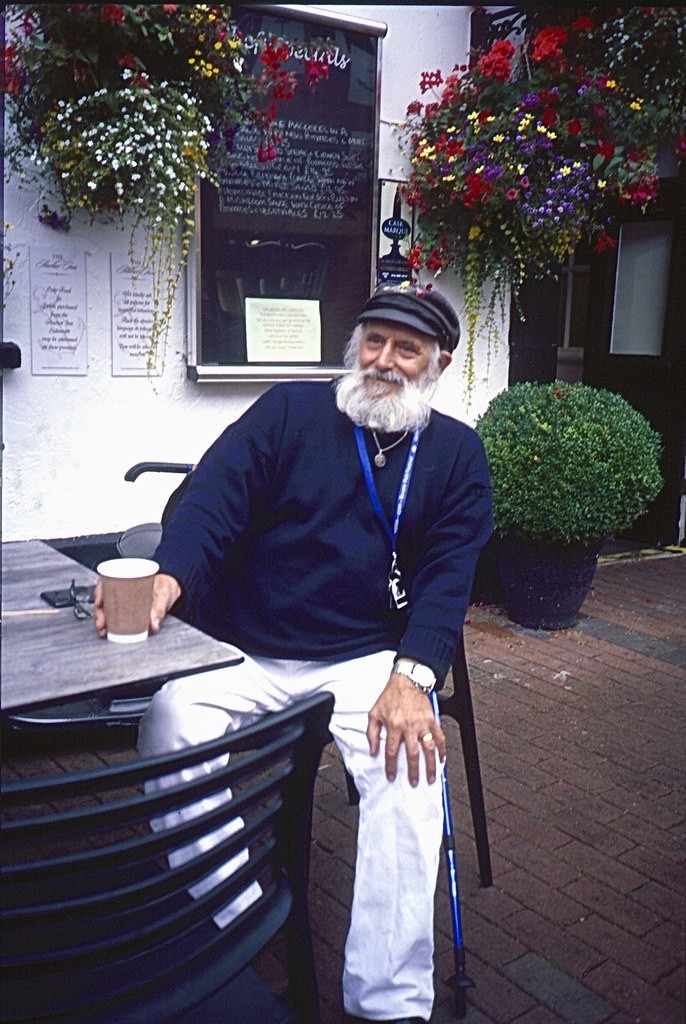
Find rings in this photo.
[418,732,433,743]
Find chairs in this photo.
[1,692,336,1023]
[116,462,493,889]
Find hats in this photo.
[356,283,463,360]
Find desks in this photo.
[1,538,245,713]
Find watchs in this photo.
[391,657,437,696]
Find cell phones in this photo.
[39,585,99,608]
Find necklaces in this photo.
[369,426,409,468]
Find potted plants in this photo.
[473,381,664,630]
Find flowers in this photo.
[390,2,686,415]
[5,0,335,394]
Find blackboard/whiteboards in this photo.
[182,4,390,383]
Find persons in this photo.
[89,280,493,1023]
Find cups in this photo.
[96,557,159,643]
